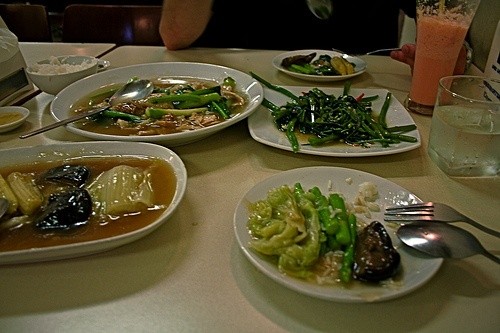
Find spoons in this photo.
[396,221,500,265]
[17,79,154,139]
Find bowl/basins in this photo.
[25,55,99,96]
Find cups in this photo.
[402,0,482,116]
[425,75,500,179]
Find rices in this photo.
[30,56,97,75]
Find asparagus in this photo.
[293,183,356,283]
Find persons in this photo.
[160,0,474,81]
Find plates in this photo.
[50,61,264,147]
[248,86,421,157]
[98,59,110,72]
[271,49,367,82]
[0,106,31,133]
[233,166,444,303]
[0,142,187,266]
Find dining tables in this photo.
[0,38,500,333]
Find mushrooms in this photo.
[351,220,402,284]
[32,163,94,237]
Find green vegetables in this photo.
[289,55,356,75]
[249,71,417,151]
[91,77,236,120]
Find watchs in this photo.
[462,39,473,74]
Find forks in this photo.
[384,201,500,238]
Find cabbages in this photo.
[0,164,157,231]
[246,185,327,282]
